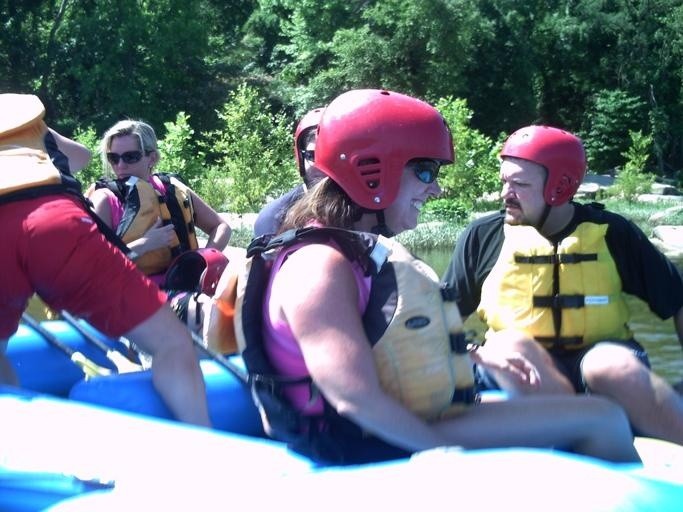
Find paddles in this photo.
[22,314,112,382]
[62,311,141,375]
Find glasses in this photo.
[106,149,156,165]
[412,161,438,183]
[300,149,315,162]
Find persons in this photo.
[439,124,683,447]
[84,118,237,369]
[0,91,238,428]
[252,91,641,464]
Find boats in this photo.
[0,322,683,512]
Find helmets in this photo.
[163,247,229,297]
[500,125,586,206]
[294,108,326,177]
[313,89,454,210]
